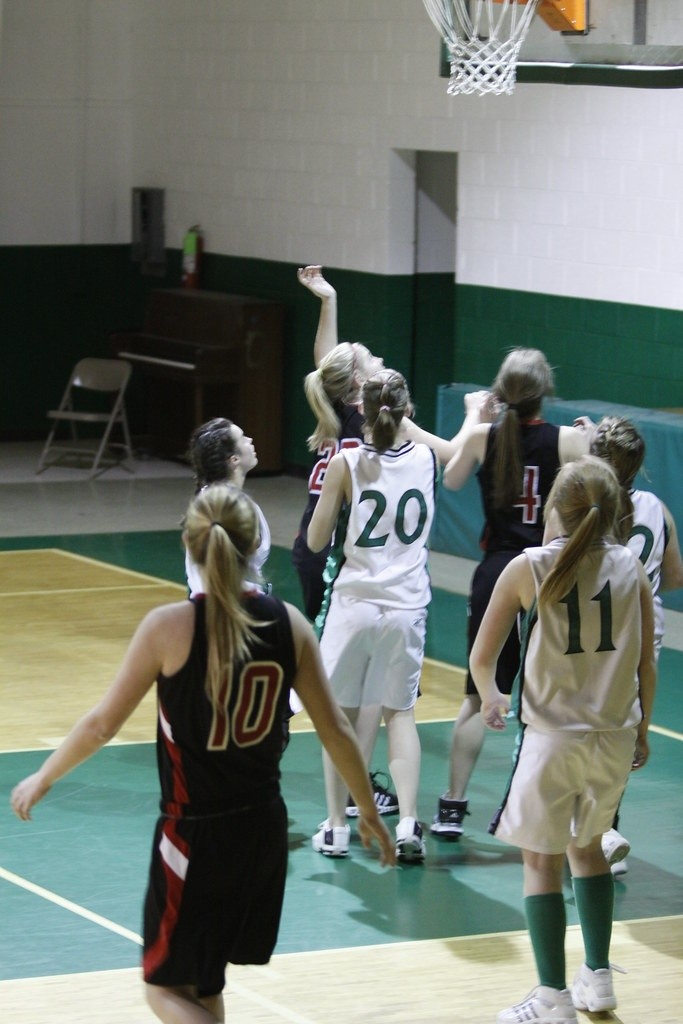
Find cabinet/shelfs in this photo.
[115,288,288,477]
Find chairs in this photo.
[34,357,136,481]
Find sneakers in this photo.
[344,771,400,817]
[610,858,629,875]
[570,961,628,1013]
[311,816,351,857]
[495,984,579,1024]
[602,826,631,867]
[395,816,427,864]
[429,797,471,837]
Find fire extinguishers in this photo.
[180,224,204,289]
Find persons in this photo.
[429,346,599,838]
[181,416,273,597]
[10,484,397,1024]
[541,415,683,877]
[468,461,655,1024]
[292,264,494,817]
[304,370,441,863]
